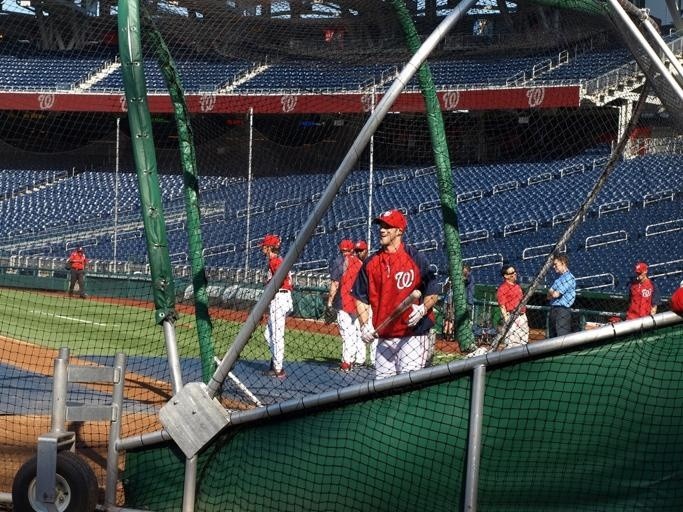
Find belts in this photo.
[276,289,288,293]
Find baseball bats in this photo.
[371,289,421,334]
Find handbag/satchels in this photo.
[64,258,71,269]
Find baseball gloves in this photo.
[324,306,338,324]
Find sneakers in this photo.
[436,333,456,341]
[262,357,285,378]
[330,362,376,374]
[69,291,88,298]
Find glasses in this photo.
[378,224,390,229]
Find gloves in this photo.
[360,324,379,343]
[408,303,428,328]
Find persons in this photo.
[259,234,294,378]
[324,239,371,372]
[65,246,90,299]
[546,255,576,339]
[496,265,529,345]
[624,262,660,321]
[671,280,683,317]
[442,265,475,342]
[354,240,378,368]
[352,210,442,381]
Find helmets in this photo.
[77,247,85,252]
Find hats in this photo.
[256,235,280,248]
[339,239,368,253]
[634,262,649,273]
[374,209,407,231]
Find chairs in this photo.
[0,30,683,316]
